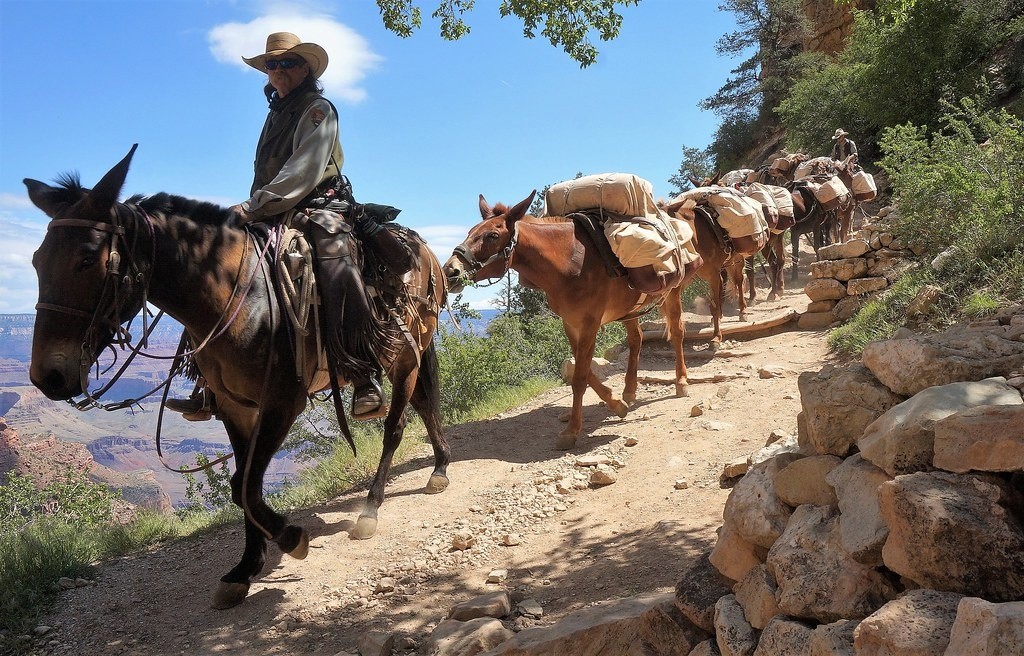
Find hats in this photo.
[241,32,329,80]
[831,128,849,140]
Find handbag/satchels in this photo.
[731,227,770,255]
[853,170,877,202]
[746,181,796,235]
[816,175,851,211]
[625,254,705,295]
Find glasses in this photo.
[264,58,303,70]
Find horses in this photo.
[22,143,449,610]
[441,151,860,449]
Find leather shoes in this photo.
[353,372,385,415]
[163,387,225,421]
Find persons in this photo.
[832,127,862,174]
[163,32,383,417]
